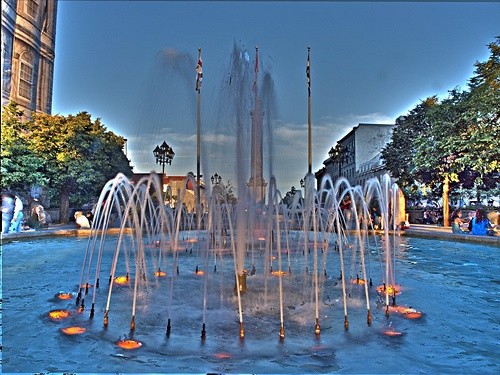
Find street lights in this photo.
[152,140,175,174]
[210,173,223,186]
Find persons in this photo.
[423,209,438,225]
[74,211,99,228]
[373,207,379,219]
[341,194,351,229]
[448,202,492,235]
[0,191,23,235]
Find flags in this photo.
[254,54,258,85]
[306,53,310,96]
[196,56,203,94]
